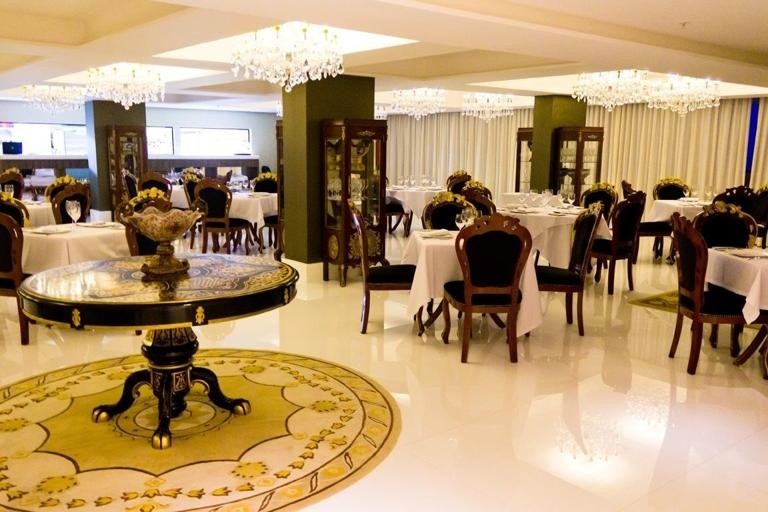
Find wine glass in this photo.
[682,185,732,204]
[397,175,437,190]
[162,173,184,188]
[517,184,575,211]
[65,198,82,234]
[328,179,369,199]
[560,147,596,162]
[225,176,256,197]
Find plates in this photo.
[422,232,453,239]
[732,248,768,259]
[504,206,584,215]
[33,228,69,234]
[85,224,115,228]
[680,198,699,202]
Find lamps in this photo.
[227,24,342,93]
[373,105,389,121]
[23,82,84,114]
[569,68,721,114]
[391,89,442,119]
[83,67,165,110]
[460,91,514,121]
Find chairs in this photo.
[420,193,486,317]
[524,198,603,336]
[1,167,92,234]
[668,213,767,378]
[0,213,52,346]
[441,213,532,364]
[121,169,279,256]
[446,168,767,296]
[384,194,412,233]
[689,202,758,358]
[349,208,432,336]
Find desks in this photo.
[15,251,299,452]
[19,221,131,277]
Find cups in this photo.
[5,185,13,199]
[454,210,484,233]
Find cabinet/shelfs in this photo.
[549,126,603,202]
[107,124,146,220]
[322,119,391,286]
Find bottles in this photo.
[31,185,37,201]
[335,154,342,169]
